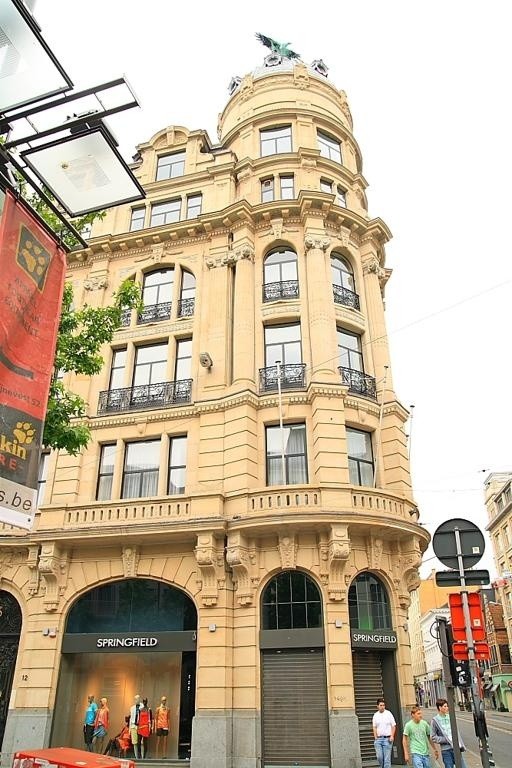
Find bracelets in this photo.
[433,749,438,753]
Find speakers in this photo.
[49,627,56,637]
[335,618,343,628]
[43,627,48,635]
[209,623,215,632]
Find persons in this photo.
[114,724,130,758]
[136,696,154,760]
[129,695,145,759]
[371,697,397,768]
[430,697,468,768]
[402,706,439,768]
[82,693,99,752]
[91,697,110,755]
[103,714,130,757]
[154,696,172,759]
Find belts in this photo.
[377,736,390,738]
